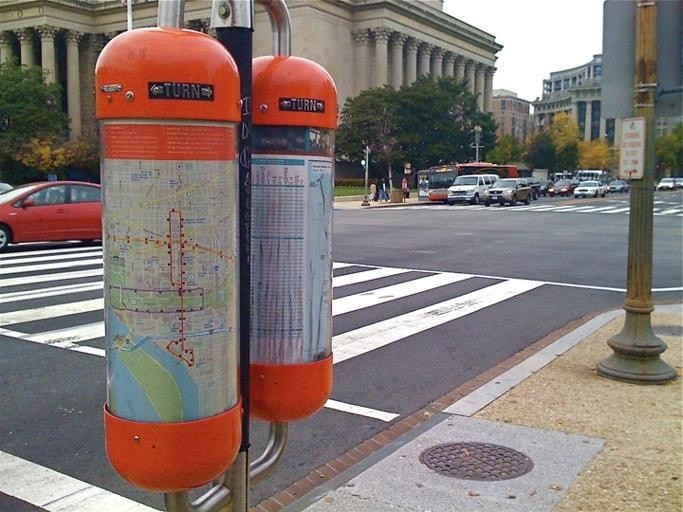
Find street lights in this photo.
[473,124,482,162]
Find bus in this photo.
[576,169,608,181]
[427,162,519,204]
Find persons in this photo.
[377,177,388,201]
[369,183,376,201]
[48,190,61,202]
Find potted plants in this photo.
[392,188,403,202]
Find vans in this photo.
[447,174,501,206]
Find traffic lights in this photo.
[360,149,367,170]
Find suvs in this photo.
[483,178,535,207]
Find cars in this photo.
[656,176,683,191]
[0,180,105,252]
[526,177,631,200]
